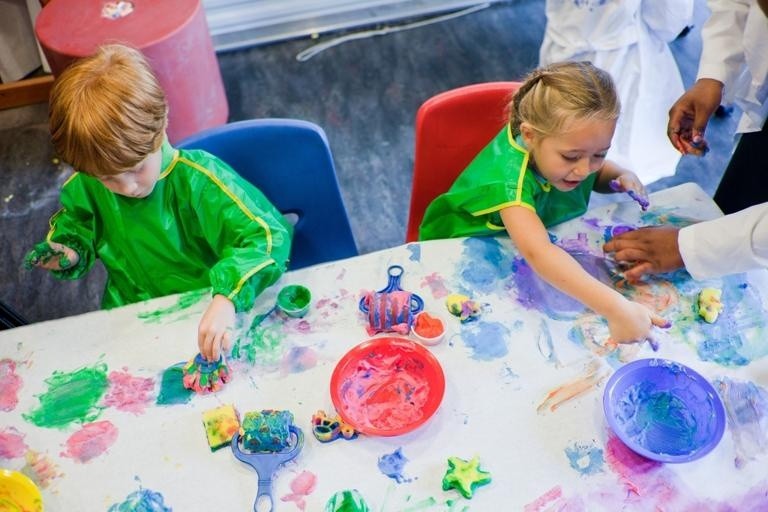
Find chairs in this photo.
[171,118,360,271]
[404,81,525,243]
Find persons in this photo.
[601,0,768,283]
[536,1,695,185]
[21,44,292,364]
[416,60,671,352]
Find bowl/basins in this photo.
[602,359,727,463]
[330,337,446,436]
[518,253,618,319]
